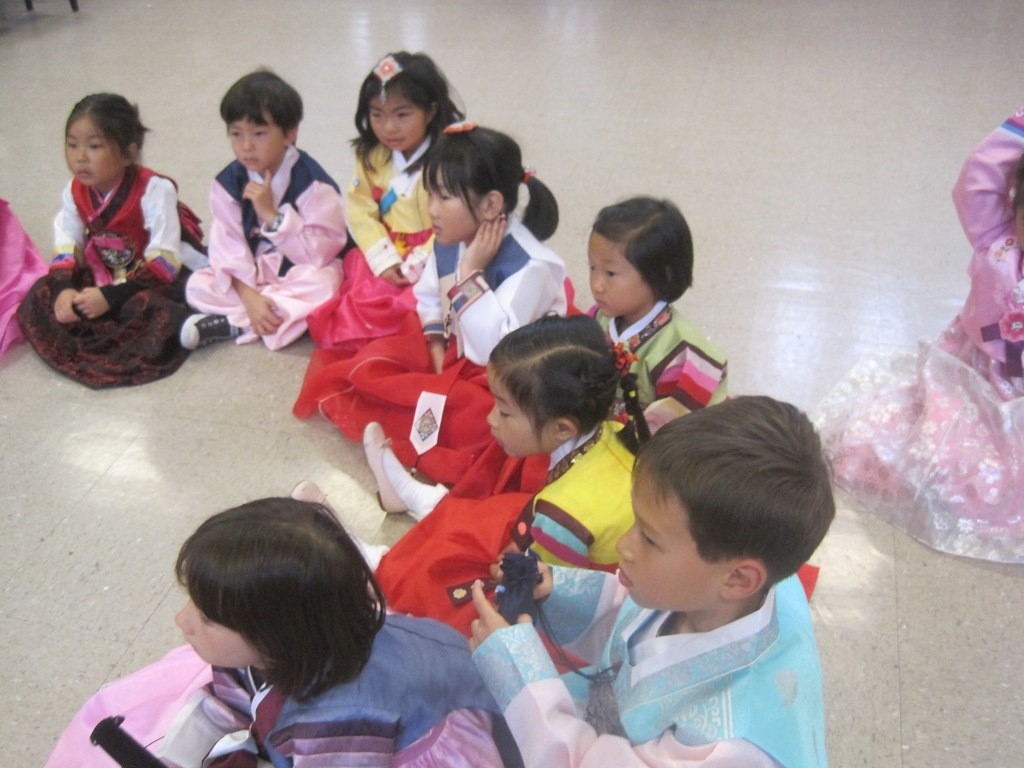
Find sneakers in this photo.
[179,313,238,350]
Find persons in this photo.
[890,110,1023,566]
[157,494,527,767]
[469,393,838,767]
[14,93,208,390]
[283,312,651,647]
[177,64,358,351]
[315,121,580,475]
[309,50,466,351]
[460,195,733,493]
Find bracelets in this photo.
[272,213,283,232]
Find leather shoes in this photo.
[291,479,342,526]
[362,421,409,514]
